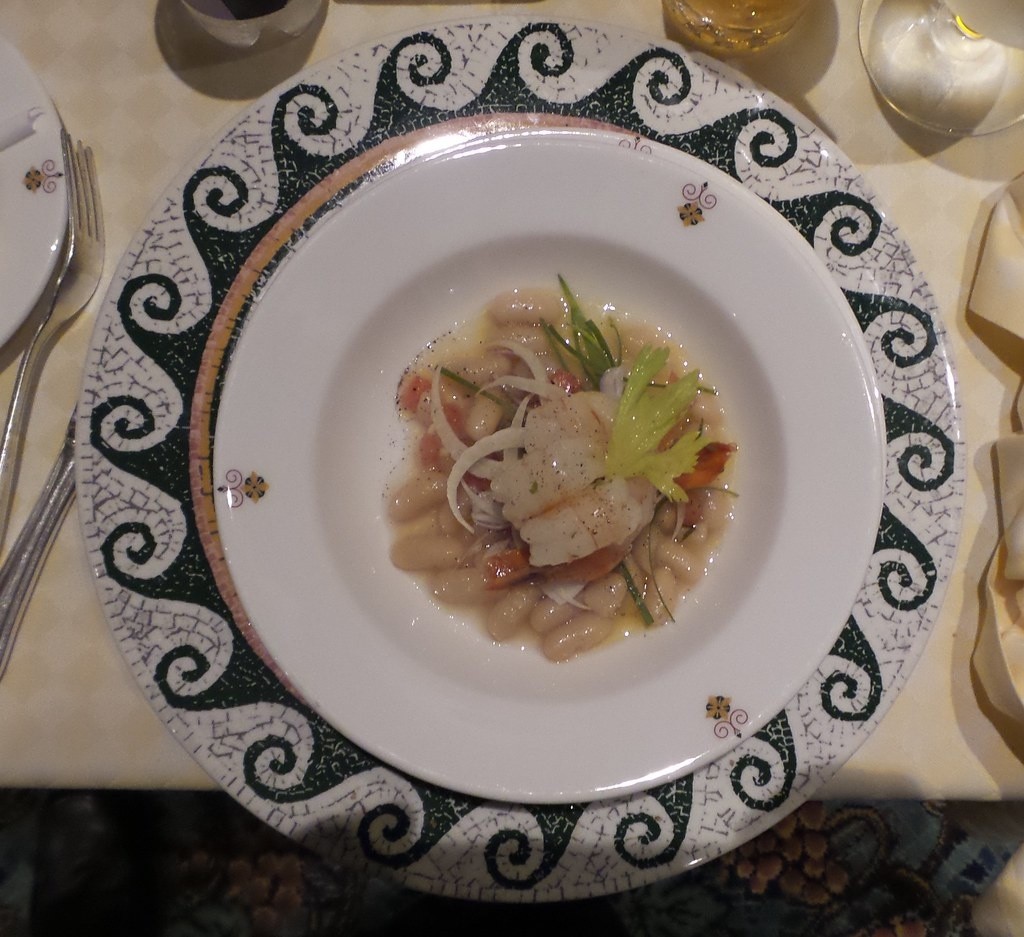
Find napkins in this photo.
[967,174,1024,718]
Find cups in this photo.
[179,0,322,49]
[665,0,809,53]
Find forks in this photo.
[0,129,107,553]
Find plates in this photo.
[0,34,69,355]
[74,16,964,907]
[213,127,888,805]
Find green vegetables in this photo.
[435,273,741,625]
